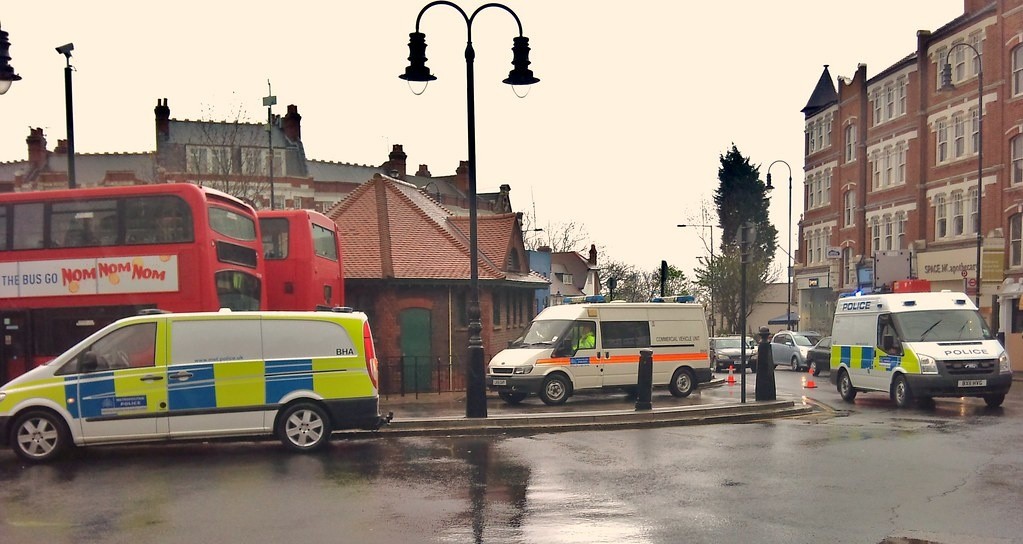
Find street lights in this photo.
[941,40,984,315]
[402,0,543,415]
[0,30,23,96]
[765,158,793,334]
[677,224,713,337]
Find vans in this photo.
[828,292,1015,409]
[1,309,392,461]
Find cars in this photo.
[769,328,821,367]
[708,337,755,372]
[809,336,832,377]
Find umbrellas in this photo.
[768,311,798,325]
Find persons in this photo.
[569,324,595,353]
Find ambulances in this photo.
[483,300,710,402]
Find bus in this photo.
[1,181,273,411]
[256,207,347,310]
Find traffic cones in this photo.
[725,365,736,383]
[803,368,818,389]
